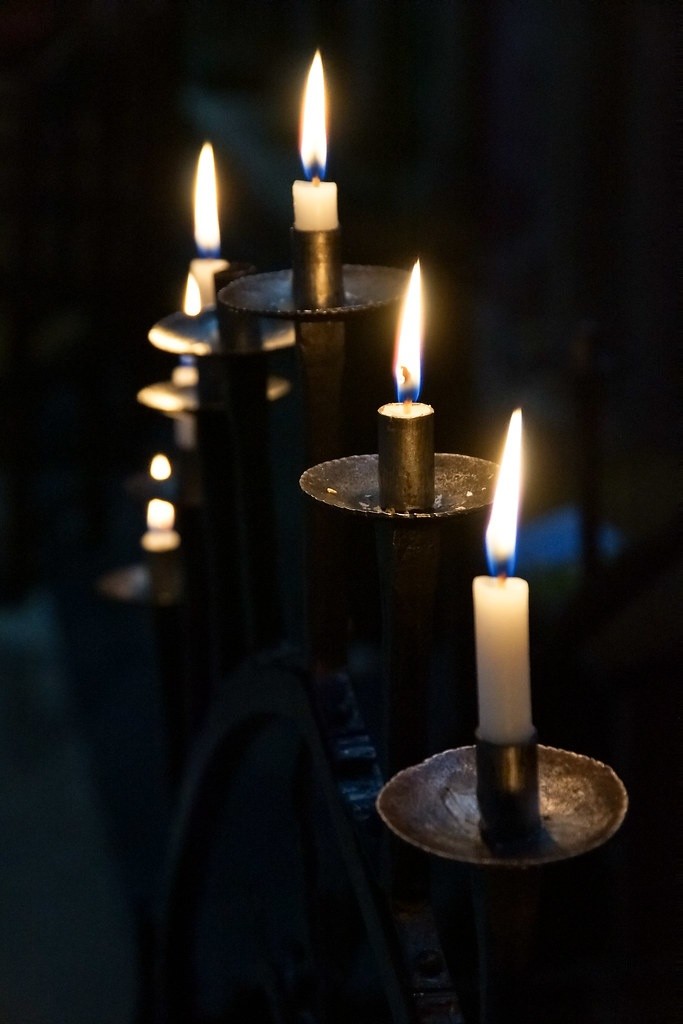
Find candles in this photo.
[141,141,229,554]
[381,258,431,419]
[292,47,338,230]
[473,406,537,746]
